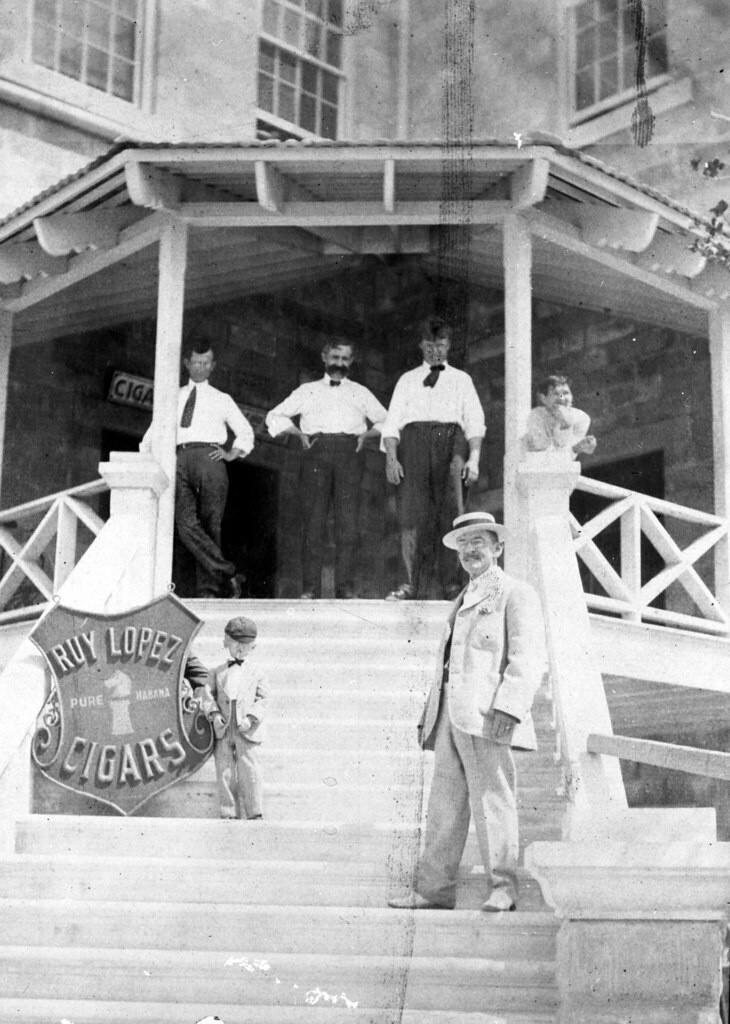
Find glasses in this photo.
[457,538,485,551]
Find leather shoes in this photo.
[196,590,213,598]
[223,572,246,599]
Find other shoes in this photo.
[484,888,516,912]
[386,588,416,600]
[335,589,358,599]
[299,591,321,599]
[387,890,453,908]
[447,588,459,600]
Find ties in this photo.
[181,387,196,427]
[463,582,477,605]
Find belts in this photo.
[443,669,450,682]
[177,442,217,451]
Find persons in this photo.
[184,616,269,818]
[265,337,388,599]
[528,375,597,455]
[139,342,255,598]
[387,512,547,911]
[379,316,487,600]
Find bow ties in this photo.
[330,380,340,386]
[424,364,445,388]
[228,658,244,667]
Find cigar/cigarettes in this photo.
[236,651,240,658]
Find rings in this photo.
[505,726,510,729]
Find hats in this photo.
[225,617,257,644]
[442,512,512,550]
